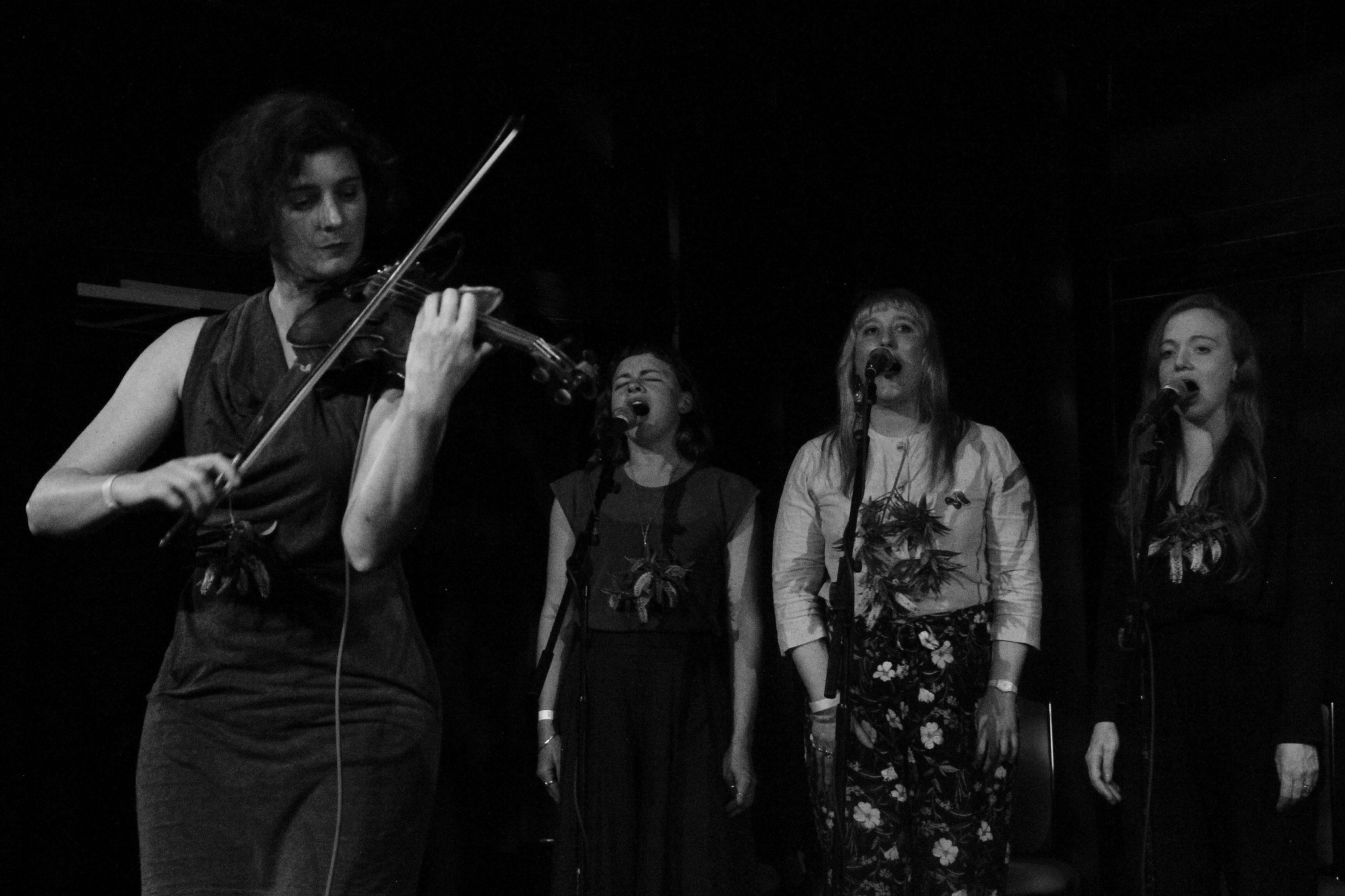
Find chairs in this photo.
[1005,699,1069,896]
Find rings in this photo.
[545,781,555,786]
[816,747,834,758]
[738,792,752,798]
[1305,784,1313,788]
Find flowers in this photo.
[193,498,277,598]
[858,488,968,611]
[598,556,692,625]
[1146,503,1249,586]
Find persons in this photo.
[770,288,1044,896]
[1086,296,1331,896]
[25,90,506,896]
[534,342,760,896]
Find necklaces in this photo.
[629,459,681,546]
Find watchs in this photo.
[988,679,1018,695]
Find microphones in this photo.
[865,346,895,376]
[599,406,637,450]
[1140,379,1189,427]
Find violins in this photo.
[286,233,602,406]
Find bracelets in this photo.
[537,709,553,720]
[103,471,133,510]
[538,733,558,751]
[809,693,840,723]
